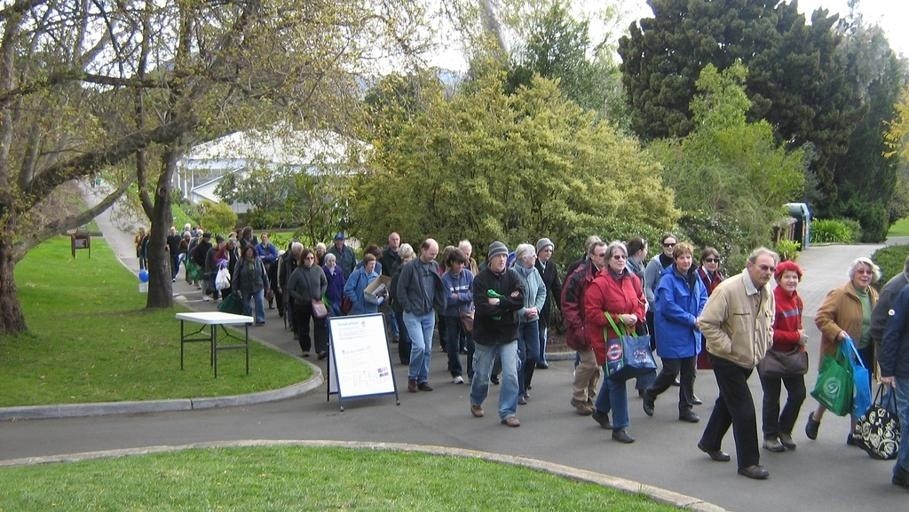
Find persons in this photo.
[269,234,389,359]
[561,233,725,443]
[167,222,277,325]
[471,237,563,426]
[382,232,479,392]
[806,257,882,445]
[698,248,809,478]
[870,258,908,487]
[135,226,151,270]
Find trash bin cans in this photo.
[784,203,810,251]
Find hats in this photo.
[536,238,555,255]
[335,232,344,240]
[487,241,509,261]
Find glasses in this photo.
[663,242,676,247]
[614,255,626,260]
[761,264,776,273]
[705,258,720,262]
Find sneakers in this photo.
[762,439,786,452]
[470,358,530,427]
[419,382,432,391]
[203,294,210,301]
[303,351,310,356]
[408,379,418,392]
[318,350,327,359]
[641,388,655,416]
[569,397,633,443]
[680,405,700,422]
[805,411,819,439]
[453,375,464,384]
[778,431,796,448]
[537,361,549,369]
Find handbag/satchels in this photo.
[459,306,476,333]
[811,353,872,419]
[310,297,327,320]
[850,404,901,460]
[215,267,231,290]
[603,311,657,382]
[340,296,352,315]
[216,290,246,315]
[758,342,808,380]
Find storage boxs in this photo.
[361,275,392,306]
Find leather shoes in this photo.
[738,463,769,478]
[697,439,730,461]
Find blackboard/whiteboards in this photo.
[330,313,395,398]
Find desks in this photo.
[174,311,255,378]
